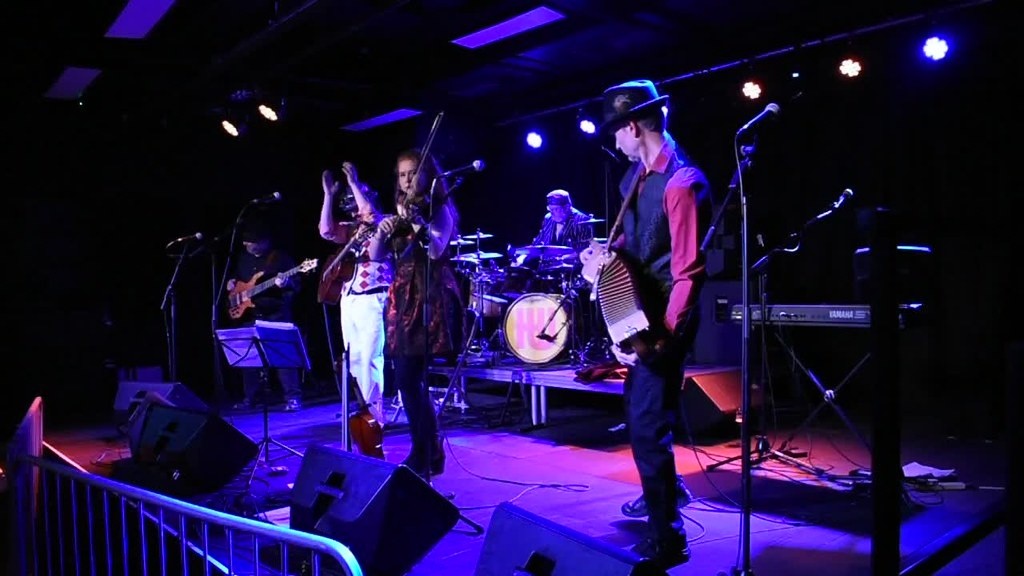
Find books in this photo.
[217,318,313,370]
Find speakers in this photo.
[677,365,765,443]
[114,379,259,505]
[686,280,743,366]
[288,442,461,576]
[471,500,671,576]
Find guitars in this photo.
[225,258,318,320]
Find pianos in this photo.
[729,304,910,499]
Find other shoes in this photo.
[397,448,447,480]
[283,399,305,412]
[232,398,262,413]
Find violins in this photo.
[317,218,376,305]
[384,174,465,254]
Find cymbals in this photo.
[519,244,575,255]
[462,234,494,239]
[543,263,574,270]
[450,251,504,265]
[449,240,476,246]
[576,218,606,225]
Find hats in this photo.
[546,190,571,205]
[593,78,672,136]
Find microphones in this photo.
[833,189,853,212]
[536,333,550,340]
[248,192,283,208]
[436,160,485,180]
[739,103,781,134]
[175,232,203,244]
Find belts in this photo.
[345,285,390,296]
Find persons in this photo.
[366,149,465,477]
[316,161,393,430]
[590,80,714,569]
[226,228,303,413]
[523,189,593,354]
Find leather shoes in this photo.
[621,473,694,518]
[631,545,692,576]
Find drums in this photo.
[501,293,577,364]
[468,292,508,320]
[593,237,608,244]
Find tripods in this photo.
[217,334,312,489]
[524,278,612,375]
[706,204,838,482]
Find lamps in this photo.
[257,85,288,121]
[221,102,251,137]
